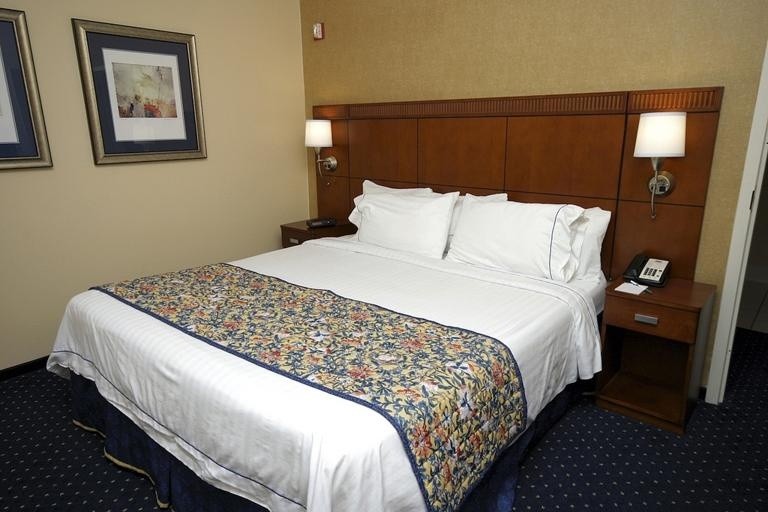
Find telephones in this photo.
[623,254,672,288]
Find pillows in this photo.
[348,179,611,284]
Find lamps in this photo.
[634,112,686,220]
[305,120,338,186]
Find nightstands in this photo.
[595,276,717,436]
[280,220,358,248]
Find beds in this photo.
[46,235,611,512]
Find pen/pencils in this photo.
[630,280,653,294]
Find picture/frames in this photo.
[0,8,53,170]
[71,18,208,165]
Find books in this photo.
[615,282,649,296]
